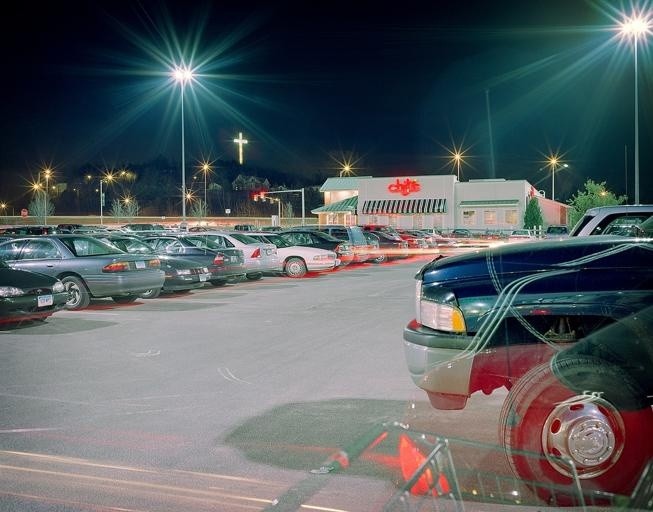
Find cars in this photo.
[547,224,568,239]
[401,231,426,248]
[450,228,472,239]
[510,229,530,241]
[567,205,653,237]
[363,231,408,263]
[0,234,165,310]
[128,234,246,288]
[272,230,355,269]
[236,231,338,278]
[412,232,433,248]
[167,231,279,283]
[0,258,69,326]
[88,234,212,298]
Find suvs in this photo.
[403,216,652,506]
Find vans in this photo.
[292,224,369,264]
